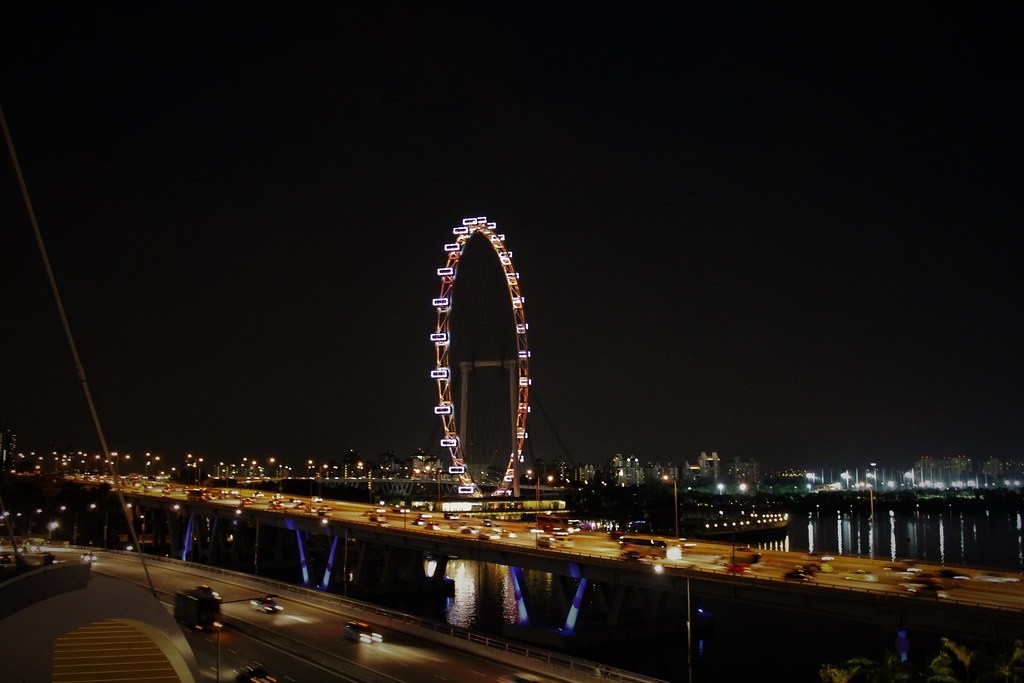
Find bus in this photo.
[22,538,44,553]
[619,535,673,559]
[175,585,222,634]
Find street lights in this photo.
[308,459,313,514]
[662,472,682,563]
[13,451,295,490]
[527,467,552,548]
[216,592,282,683]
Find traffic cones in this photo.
[357,461,372,521]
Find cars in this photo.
[80,473,580,551]
[0,554,11,563]
[715,547,762,574]
[250,596,279,614]
[82,551,97,563]
[784,553,835,585]
[345,621,379,643]
[841,559,1021,601]
